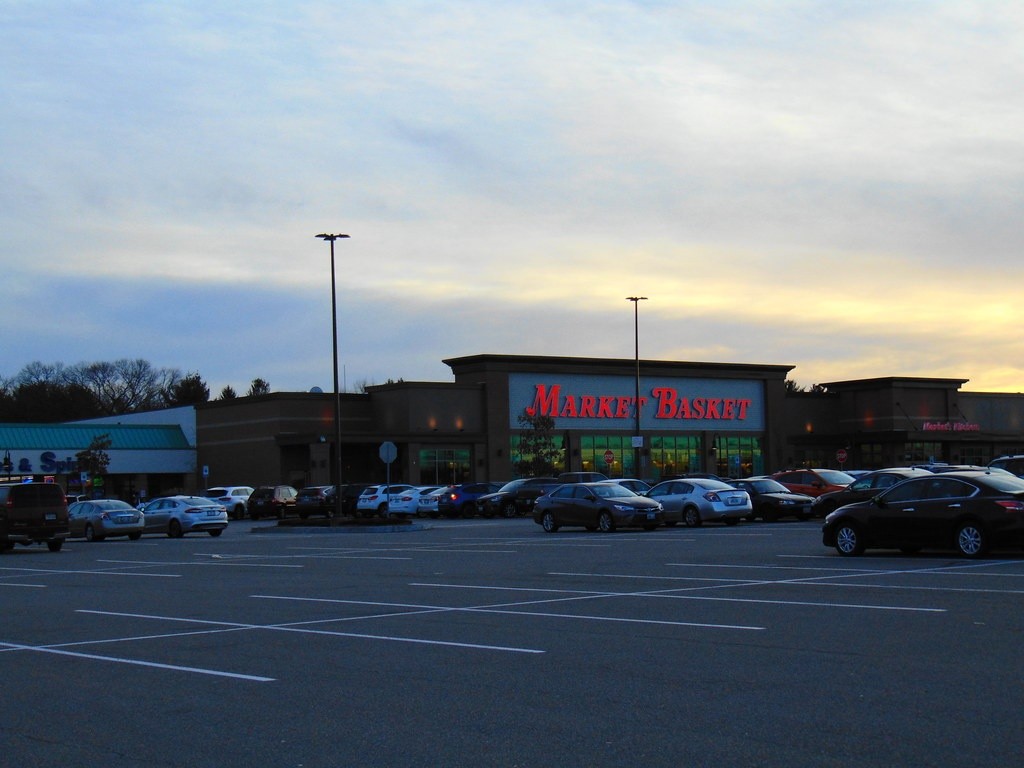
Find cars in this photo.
[202,486,254,520]
[908,454,1024,480]
[140,495,229,539]
[532,482,665,534]
[417,484,462,519]
[810,466,935,519]
[356,483,412,520]
[723,479,816,522]
[821,471,1024,559]
[436,482,505,518]
[598,478,650,497]
[843,470,871,486]
[388,486,441,520]
[296,486,336,517]
[473,477,557,519]
[323,483,376,515]
[68,499,145,543]
[767,468,857,496]
[643,477,753,528]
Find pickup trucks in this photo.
[515,473,609,516]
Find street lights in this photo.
[314,232,353,513]
[625,296,650,475]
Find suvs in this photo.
[0,480,72,552]
[246,484,299,519]
